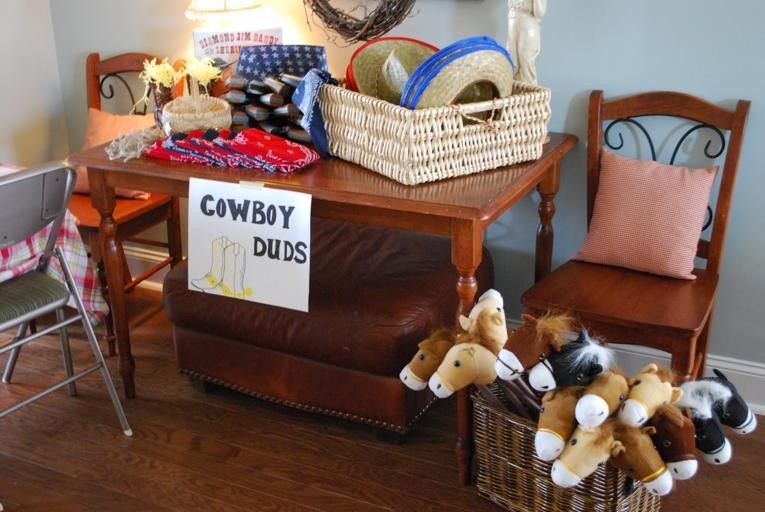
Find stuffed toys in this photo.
[399,287,757,496]
[400,287,757,495]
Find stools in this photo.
[162,217,494,444]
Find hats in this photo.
[343,38,513,111]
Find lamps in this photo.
[184,0,266,17]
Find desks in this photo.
[67,131,579,489]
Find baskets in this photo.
[162,75,233,137]
[316,76,552,188]
[469,381,663,512]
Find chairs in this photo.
[522,88,751,388]
[68,51,186,356]
[0,160,134,436]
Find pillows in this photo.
[570,145,721,281]
